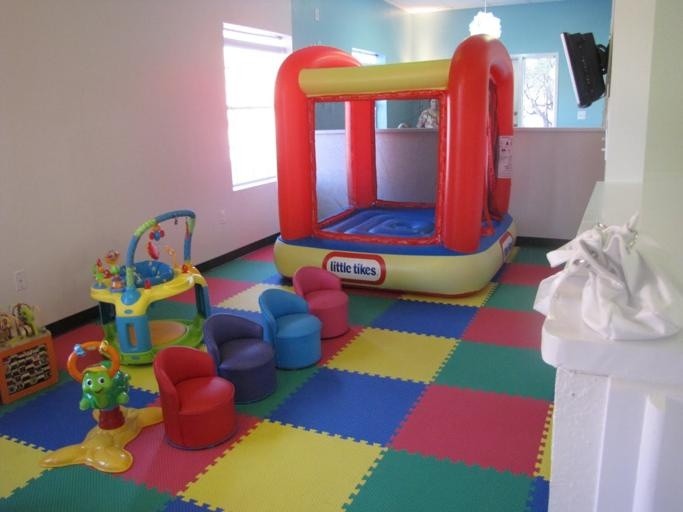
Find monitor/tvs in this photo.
[560,32,606,107]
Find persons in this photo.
[415,98,439,128]
